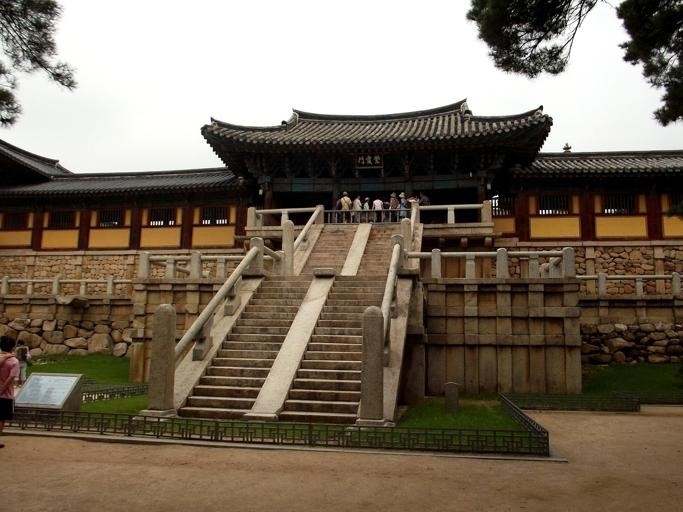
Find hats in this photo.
[398,192,406,198]
[390,192,396,197]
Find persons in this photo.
[335,191,430,223]
[13,339,31,387]
[0,335,20,449]
